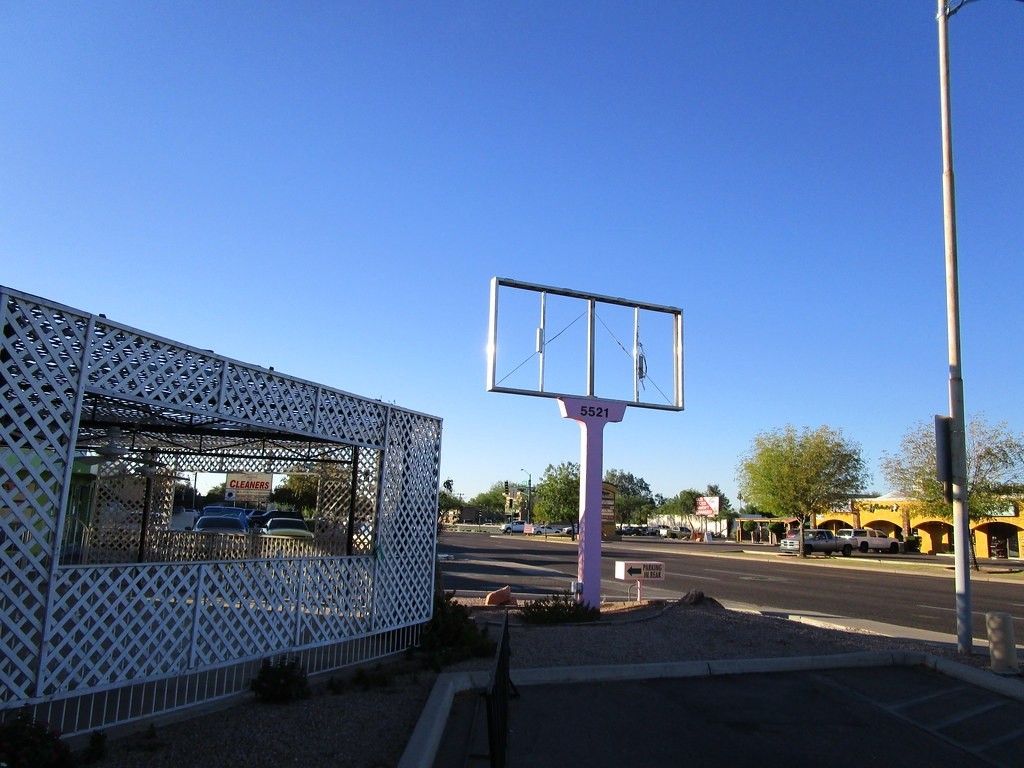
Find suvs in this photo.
[836,528,901,554]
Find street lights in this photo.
[521,468,532,522]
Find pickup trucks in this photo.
[780,529,859,557]
[659,526,692,540]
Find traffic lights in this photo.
[504,481,510,495]
[509,499,512,509]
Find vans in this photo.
[254,510,305,533]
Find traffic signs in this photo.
[625,561,665,581]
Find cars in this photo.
[617,524,671,537]
[193,506,268,530]
[184,515,247,558]
[531,525,561,536]
[563,524,578,535]
[260,518,314,551]
[500,520,527,534]
